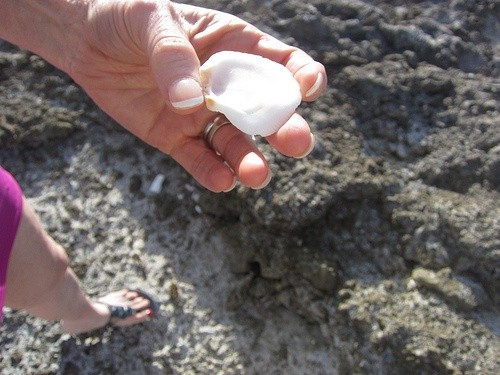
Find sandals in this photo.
[76,288,155,338]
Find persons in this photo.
[0,0,328,339]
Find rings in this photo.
[203,113,231,154]
